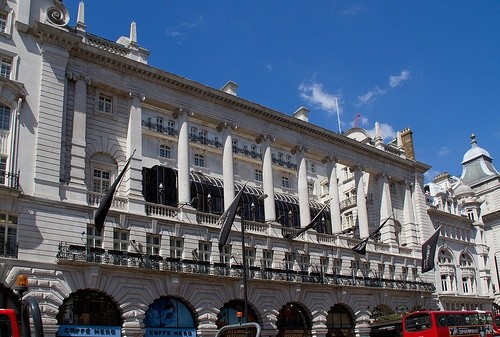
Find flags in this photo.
[217,185,246,247]
[288,205,326,239]
[94,157,132,232]
[421,227,441,274]
[352,215,392,255]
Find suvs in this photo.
[0,276,43,337]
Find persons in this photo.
[409,311,485,329]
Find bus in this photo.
[403,308,495,337]
